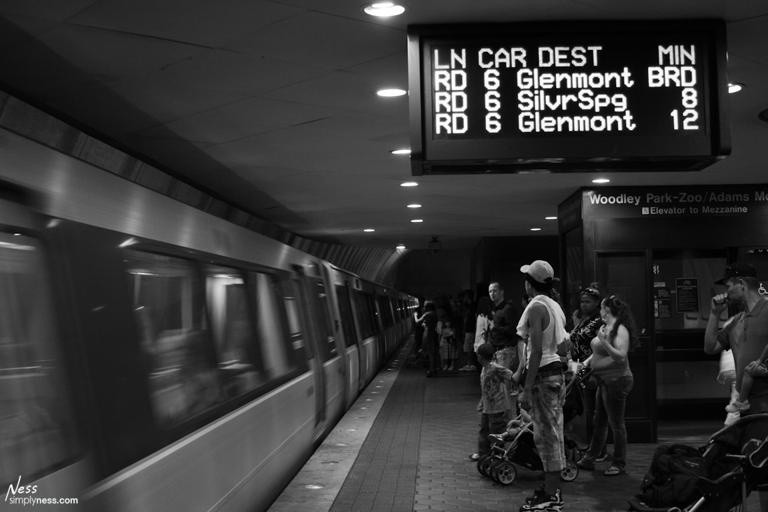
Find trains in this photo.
[0,124,412,508]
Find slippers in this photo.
[603,465,627,477]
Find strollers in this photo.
[624,412,768,511]
[475,358,594,486]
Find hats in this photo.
[520,260,555,283]
[712,262,758,284]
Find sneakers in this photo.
[577,456,597,471]
[519,489,566,511]
[724,398,751,414]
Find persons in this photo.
[704,262,768,510]
[412,258,639,511]
